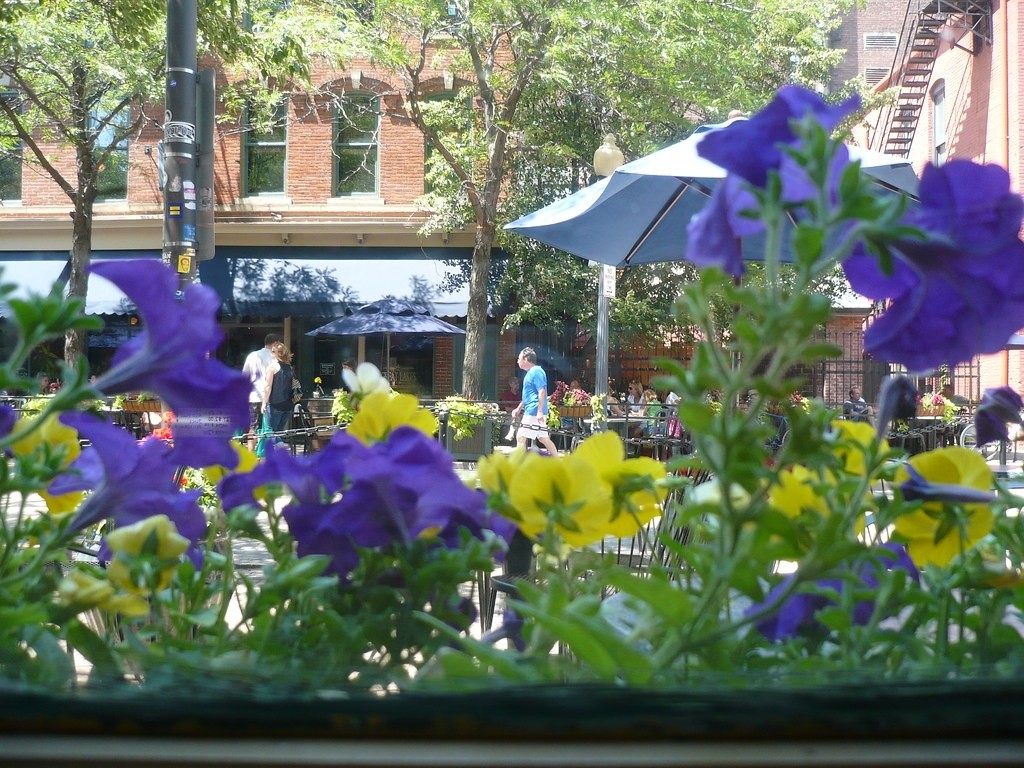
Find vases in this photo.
[914,405,945,416]
[557,407,592,416]
[313,392,320,399]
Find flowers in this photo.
[912,393,956,418]
[547,381,608,430]
[314,376,323,393]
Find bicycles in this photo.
[958,415,1024,462]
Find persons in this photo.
[935,384,970,448]
[709,388,724,403]
[241,333,304,458]
[561,375,690,462]
[502,376,522,441]
[33,370,49,395]
[332,360,361,412]
[511,347,558,458]
[742,389,774,446]
[90,374,99,384]
[56,377,65,391]
[843,387,876,429]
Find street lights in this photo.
[593,134,624,438]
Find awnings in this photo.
[0,245,517,321]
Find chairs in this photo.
[298,406,329,456]
[635,408,681,460]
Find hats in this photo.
[508,376,521,385]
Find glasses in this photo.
[628,386,636,391]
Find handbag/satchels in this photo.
[290,366,303,405]
[257,412,275,456]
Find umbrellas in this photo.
[999,328,1024,347]
[501,110,923,414]
[304,293,469,373]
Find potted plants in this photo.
[433,395,502,463]
